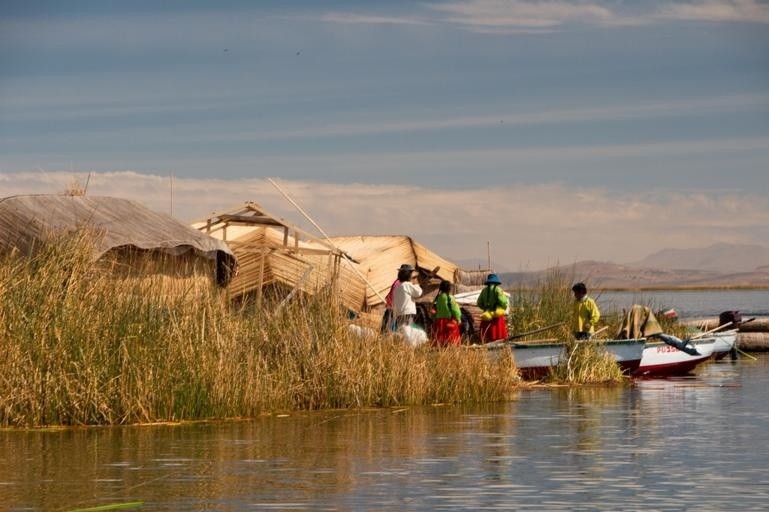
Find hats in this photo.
[484,274,502,284]
[398,264,415,271]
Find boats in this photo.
[424,337,646,382]
[631,337,715,378]
[689,332,737,361]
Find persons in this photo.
[572,283,600,340]
[476,274,507,344]
[382,265,412,333]
[431,281,461,347]
[393,270,423,328]
